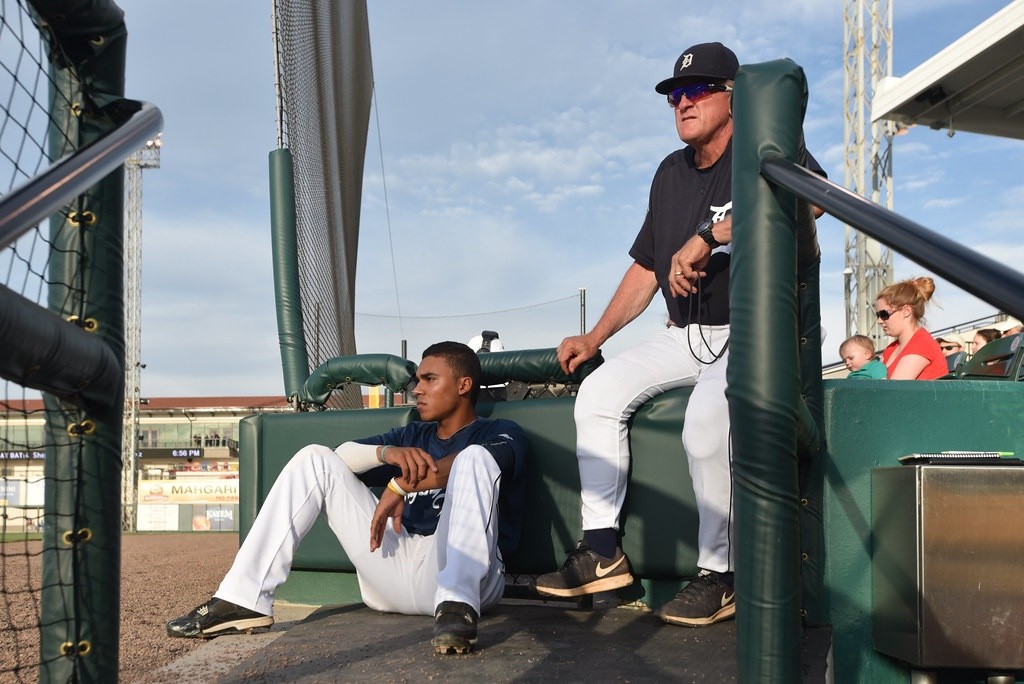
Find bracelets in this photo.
[388,477,407,496]
[382,445,396,463]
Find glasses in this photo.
[667,82,733,107]
[941,345,961,351]
[876,304,912,320]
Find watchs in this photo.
[696,219,721,249]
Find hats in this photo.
[655,42,739,95]
[991,315,1024,331]
[934,334,966,348]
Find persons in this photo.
[166,342,528,655]
[839,335,888,380]
[536,42,828,628]
[874,277,949,380]
[939,334,966,358]
[971,318,1024,355]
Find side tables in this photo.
[868,465,1024,684]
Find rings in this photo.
[674,272,682,276]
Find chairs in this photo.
[934,333,1024,382]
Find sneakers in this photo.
[430,601,478,654]
[535,540,633,597]
[166,598,274,637]
[654,568,735,627]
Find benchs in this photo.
[238,348,1024,684]
